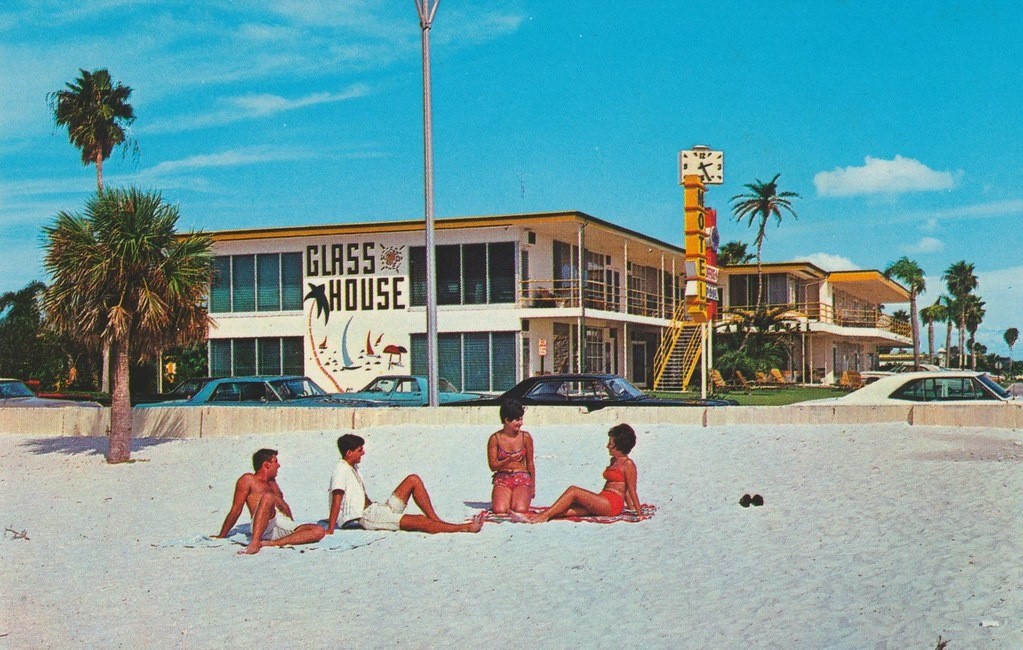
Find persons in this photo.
[320,434,485,539]
[486,402,537,513]
[520,423,644,524]
[209,448,325,554]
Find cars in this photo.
[0,378,106,408]
[328,375,483,406]
[134,375,398,408]
[146,377,244,403]
[783,370,1023,405]
[423,372,739,412]
